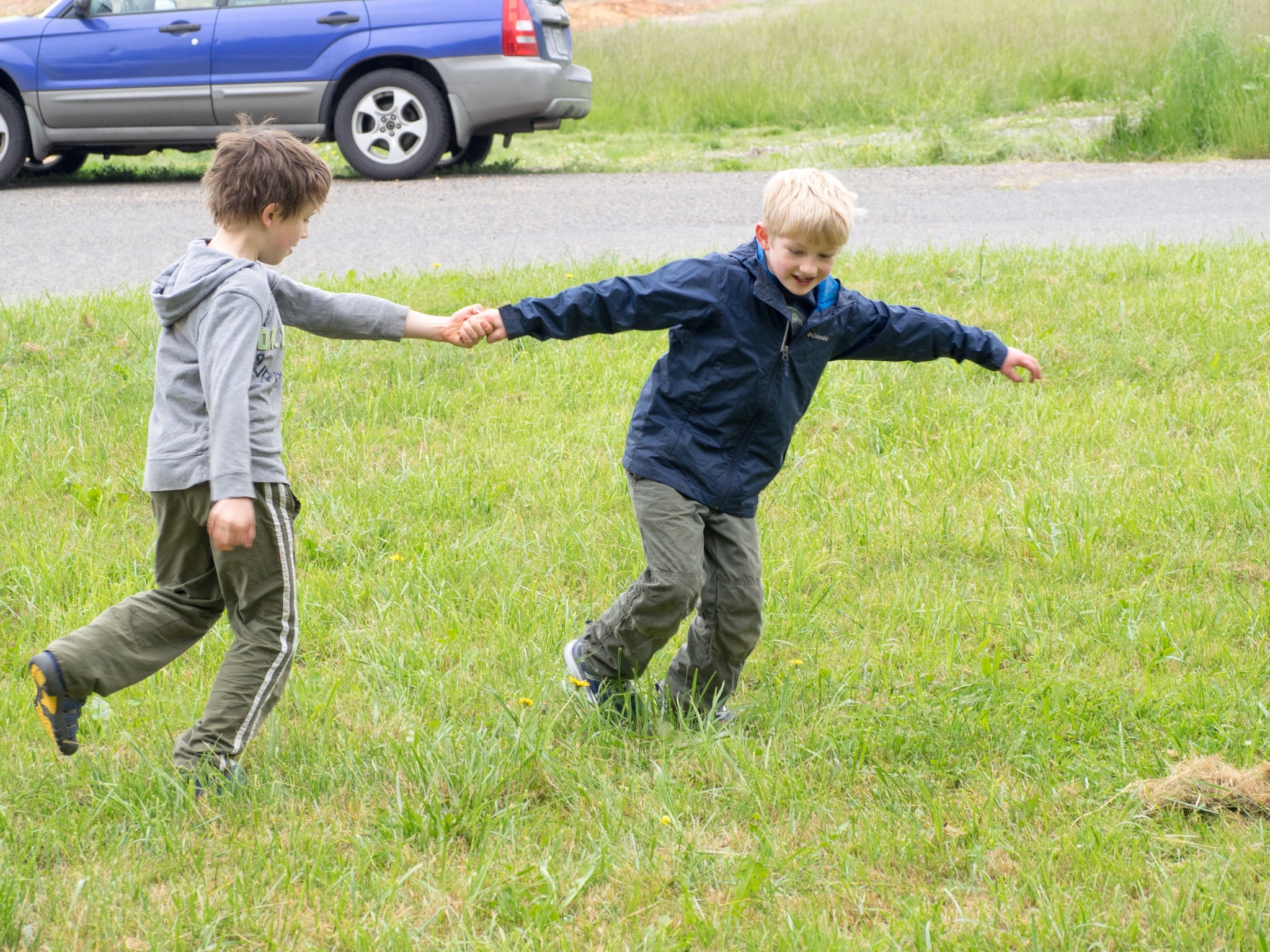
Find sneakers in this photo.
[28,652,80,756]
[183,765,250,800]
[655,682,742,730]
[565,640,649,732]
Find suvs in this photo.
[0,0,593,190]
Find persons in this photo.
[27,113,491,794]
[465,168,1043,734]
